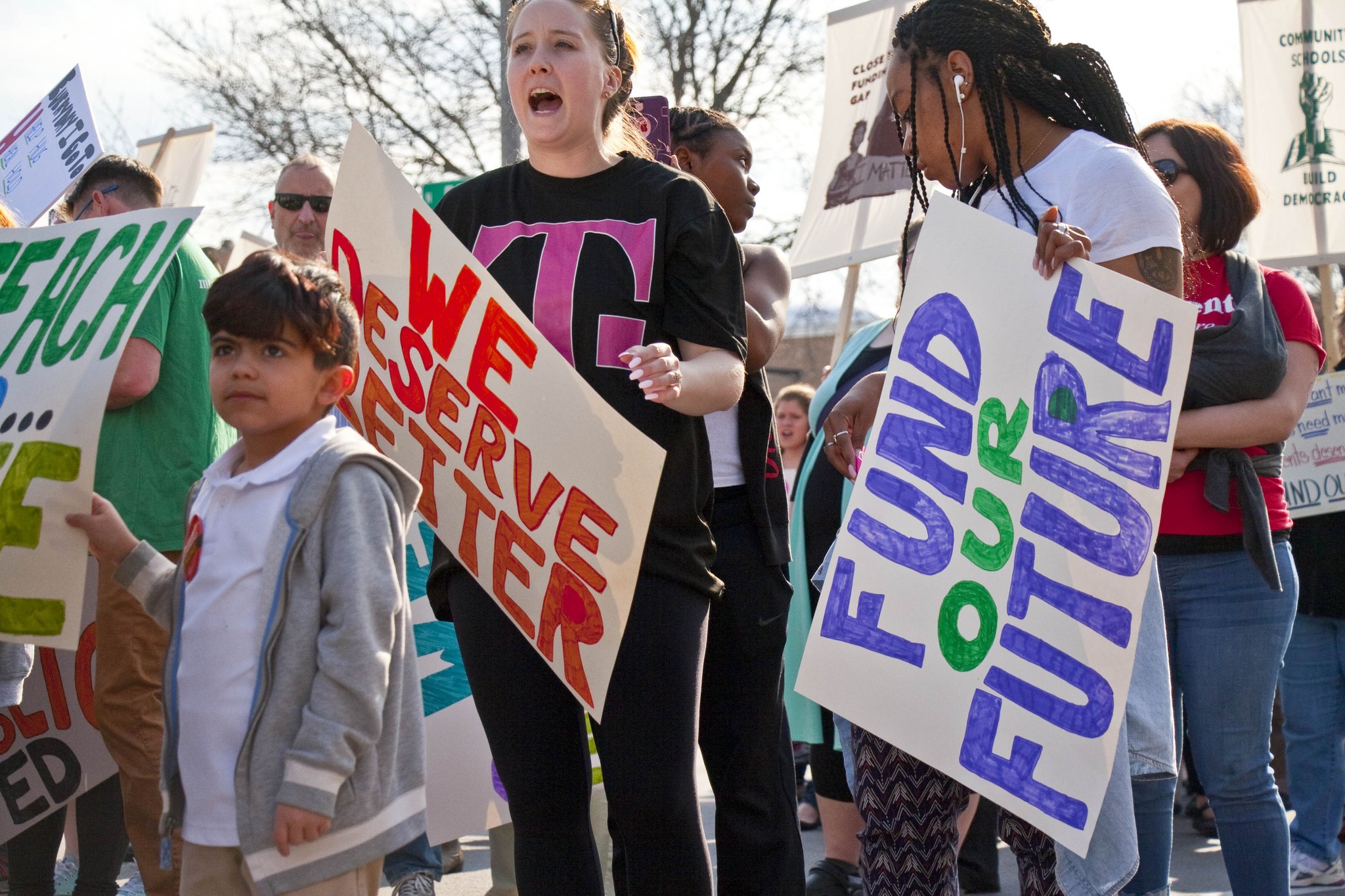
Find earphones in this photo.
[953,74,964,88]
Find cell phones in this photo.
[628,95,671,165]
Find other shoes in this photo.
[124,844,135,861]
[1184,797,1218,837]
[808,858,864,896]
[1279,793,1294,811]
[798,796,821,831]
[440,840,464,874]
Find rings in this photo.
[832,431,849,444]
[823,442,834,453]
[670,370,683,384]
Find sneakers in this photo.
[391,871,435,896]
[116,867,146,896]
[53,855,79,896]
[1290,844,1345,887]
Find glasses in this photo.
[275,193,332,213]
[1152,158,1194,188]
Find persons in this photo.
[775,0,1186,896]
[438,839,464,876]
[668,106,795,896]
[65,248,428,896]
[431,0,748,896]
[268,157,442,895]
[0,195,37,709]
[1166,276,1345,896]
[1138,118,1328,896]
[66,154,238,896]
[6,772,131,896]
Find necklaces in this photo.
[1002,124,1060,184]
